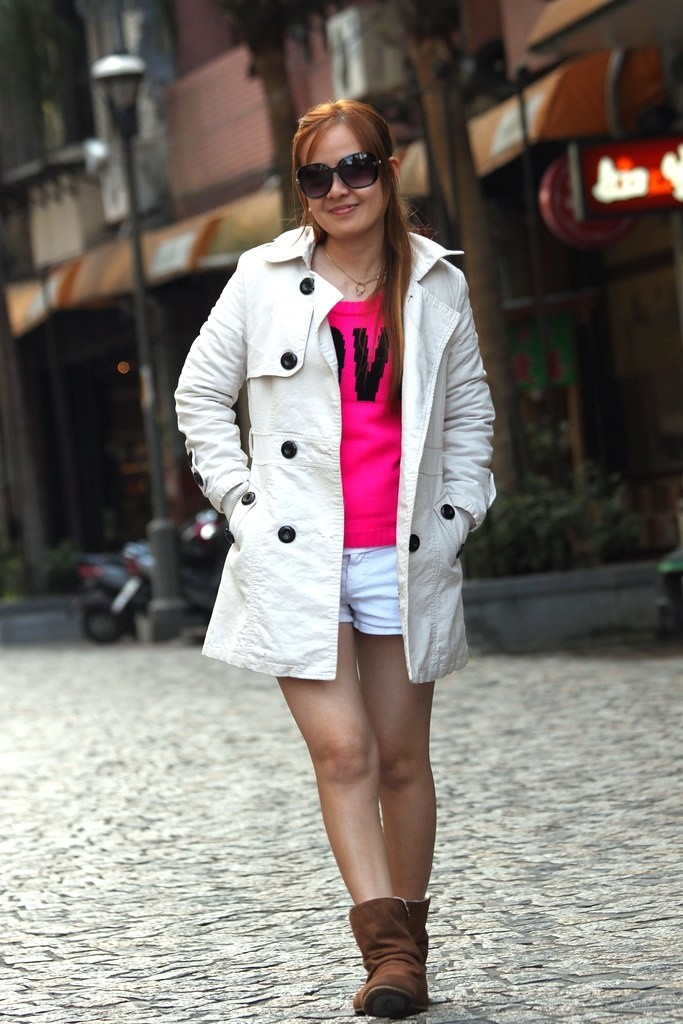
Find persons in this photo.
[173,100,496,1018]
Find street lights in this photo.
[91,50,194,644]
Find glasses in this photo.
[295,152,385,198]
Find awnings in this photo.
[9,50,660,338]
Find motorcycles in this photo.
[69,554,152,646]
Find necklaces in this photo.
[322,243,380,296]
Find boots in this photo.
[348,898,430,1017]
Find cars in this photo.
[176,510,235,627]
